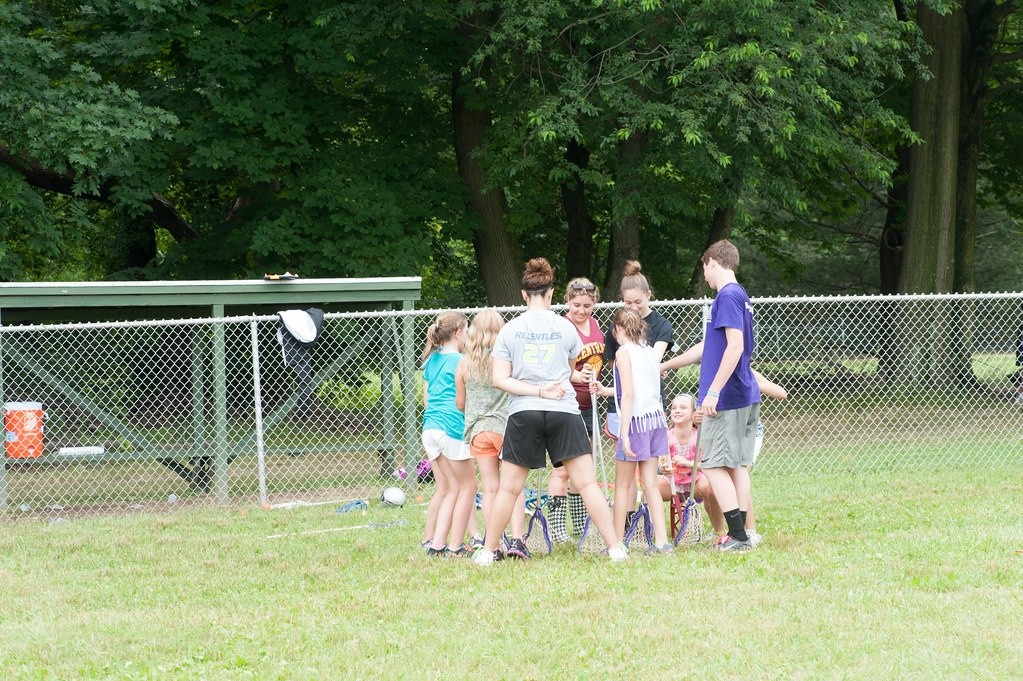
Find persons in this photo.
[421,240,787,566]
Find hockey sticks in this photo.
[672,423,701,546]
[482,530,511,551]
[666,447,684,539]
[624,490,654,549]
[524,467,551,558]
[578,367,615,560]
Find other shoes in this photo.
[421,539,449,549]
[746,530,763,546]
[719,538,750,552]
[645,543,673,555]
[708,535,732,548]
[470,533,482,549]
[474,550,494,563]
[609,548,629,561]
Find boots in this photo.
[568,491,594,536]
[547,495,578,545]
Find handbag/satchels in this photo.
[394,458,435,485]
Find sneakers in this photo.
[426,544,448,557]
[493,545,505,561]
[445,543,476,558]
[507,539,532,561]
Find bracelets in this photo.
[539,384,544,398]
[657,464,663,476]
[707,389,720,398]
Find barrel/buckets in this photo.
[4,402,48,459]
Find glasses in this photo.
[569,284,596,292]
